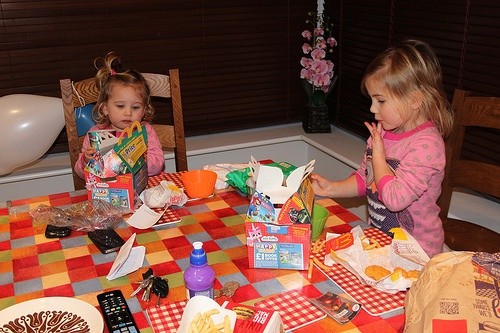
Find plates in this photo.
[0,297,104,333]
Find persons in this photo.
[310,39,454,259]
[73,52,165,180]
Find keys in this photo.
[130,275,170,307]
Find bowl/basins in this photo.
[181,170,217,198]
[311,205,329,239]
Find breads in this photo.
[365,265,421,280]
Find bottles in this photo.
[183,241,216,300]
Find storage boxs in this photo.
[243,155,315,270]
[178,295,285,333]
[84,121,147,214]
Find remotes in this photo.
[97,289,141,333]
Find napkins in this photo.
[325,225,424,296]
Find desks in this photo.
[0,158,406,333]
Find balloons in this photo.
[0,94,98,176]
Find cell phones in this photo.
[45,218,71,238]
[88,227,126,254]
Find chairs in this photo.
[59,69,189,189]
[436,88,500,254]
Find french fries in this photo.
[187,309,231,333]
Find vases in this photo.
[300,82,340,133]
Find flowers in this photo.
[299,11,338,93]
